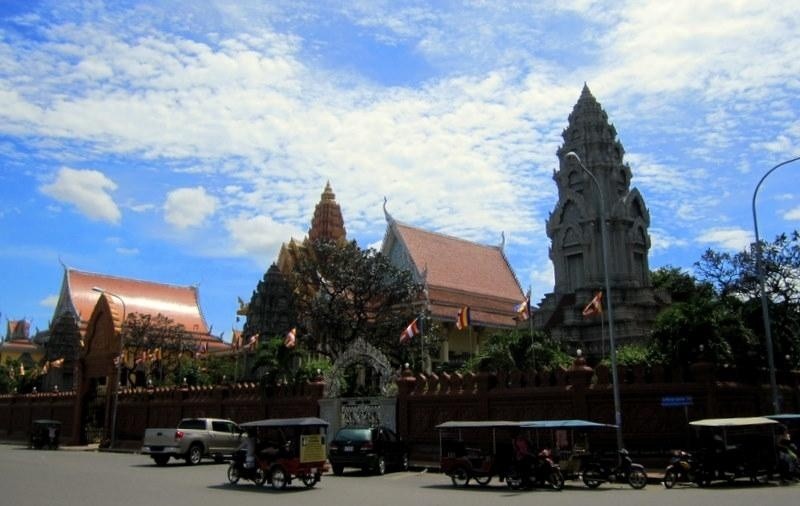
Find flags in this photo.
[232,332,240,352]
[582,291,603,322]
[399,312,423,345]
[284,327,296,349]
[243,335,257,355]
[457,302,472,329]
[6,358,65,380]
[514,288,529,322]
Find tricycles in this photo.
[436,422,648,491]
[227,417,329,492]
[664,417,781,488]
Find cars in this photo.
[328,424,411,475]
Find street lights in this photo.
[565,151,623,469]
[753,157,800,414]
[92,285,125,449]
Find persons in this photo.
[48,424,59,443]
[707,433,727,466]
[514,428,534,462]
[236,432,255,476]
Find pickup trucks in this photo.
[140,417,248,466]
